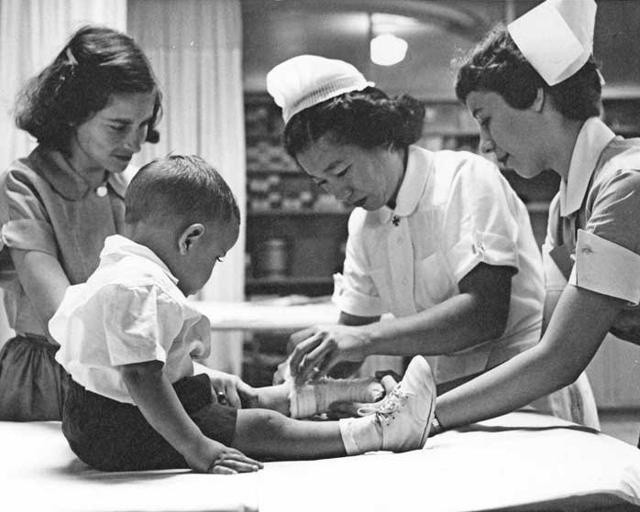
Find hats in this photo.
[265,54,375,125]
[507,0,598,87]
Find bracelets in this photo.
[432,415,442,433]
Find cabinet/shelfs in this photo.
[242,93,640,287]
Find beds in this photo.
[0,404,640,512]
[189,299,394,333]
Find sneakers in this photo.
[377,354,437,451]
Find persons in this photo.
[46,147,436,477]
[326,0,640,450]
[0,24,259,424]
[264,52,603,433]
